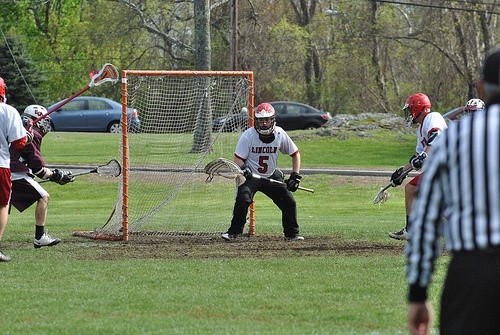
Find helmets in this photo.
[0,77,6,98]
[402,93,431,124]
[254,103,276,135]
[23,104,51,137]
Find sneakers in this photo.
[0,251,11,261]
[388,228,408,240]
[34,229,61,249]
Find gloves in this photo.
[240,164,252,180]
[284,172,302,192]
[20,116,33,133]
[47,168,76,186]
[409,155,424,171]
[390,167,408,188]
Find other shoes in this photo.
[284,234,304,241]
[221,233,242,239]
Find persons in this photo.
[388,92,486,241]
[221,104,305,241]
[406,44,500,335]
[0,77,33,262]
[8,105,75,248]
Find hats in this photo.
[482,46,500,84]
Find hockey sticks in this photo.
[203,157,314,193]
[373,152,427,205]
[34,63,118,124]
[38,158,122,184]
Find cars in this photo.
[212,101,333,133]
[20,96,141,133]
